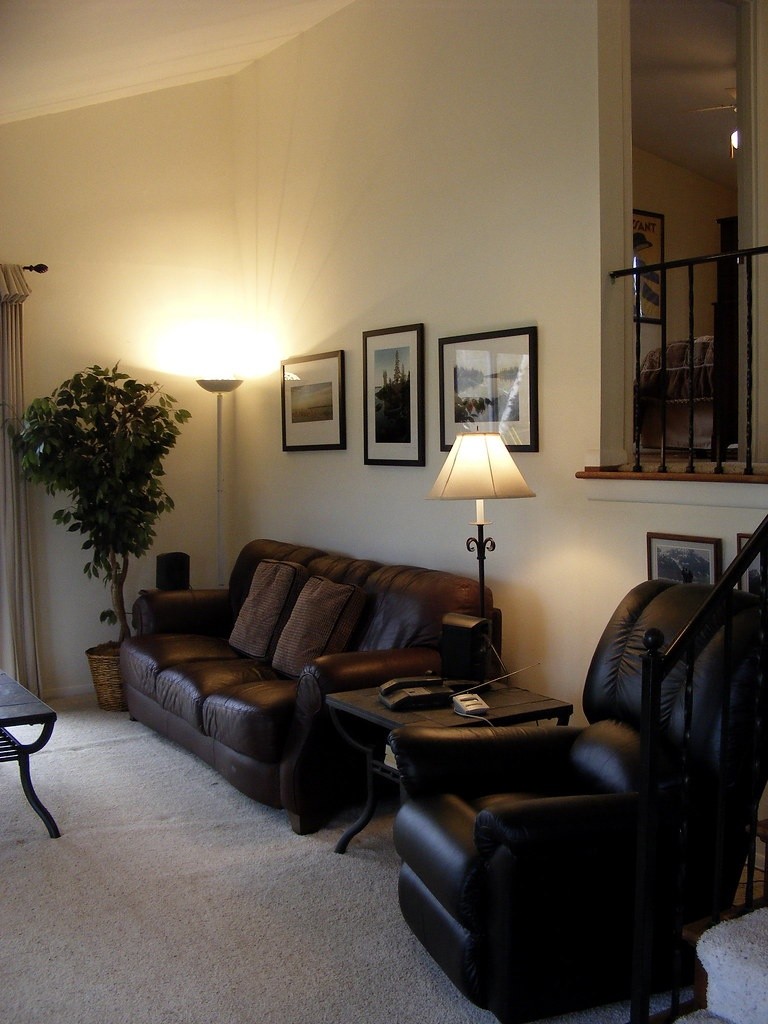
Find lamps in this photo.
[196,380,244,589]
[426,426,536,618]
[730,108,739,158]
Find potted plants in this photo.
[0,366,191,712]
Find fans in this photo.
[674,88,737,114]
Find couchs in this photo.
[119,539,502,835]
[388,580,768,1024]
[629,336,714,459]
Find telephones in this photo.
[378,675,456,709]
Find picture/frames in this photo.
[737,533,760,593]
[647,532,721,588]
[438,325,539,452]
[632,208,666,325]
[280,350,346,451]
[362,322,425,467]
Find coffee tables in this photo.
[326,680,573,854]
[0,670,61,839]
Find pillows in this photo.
[272,575,366,682]
[228,559,310,664]
